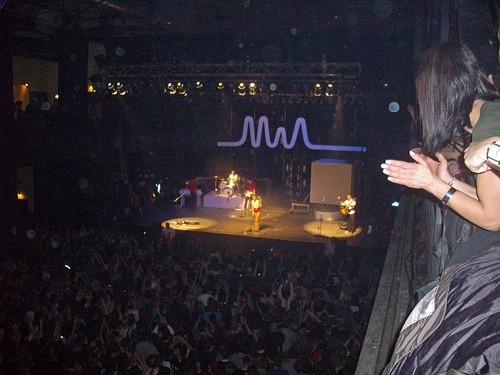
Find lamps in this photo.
[176,82,188,97]
[217,83,225,90]
[314,84,322,96]
[116,82,127,95]
[237,82,247,96]
[248,82,256,96]
[167,83,176,95]
[106,83,118,95]
[326,83,333,96]
[196,81,208,96]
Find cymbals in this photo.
[220,179,228,181]
[212,176,220,178]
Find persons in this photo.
[0,222,368,374]
[342,194,358,234]
[186,177,198,207]
[252,196,263,233]
[228,170,239,197]
[380,40,499,233]
[243,179,256,210]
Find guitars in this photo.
[340,198,356,216]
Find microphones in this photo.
[322,197,325,201]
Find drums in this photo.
[218,181,228,189]
[222,185,234,197]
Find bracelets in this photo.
[441,187,457,206]
[447,177,455,185]
